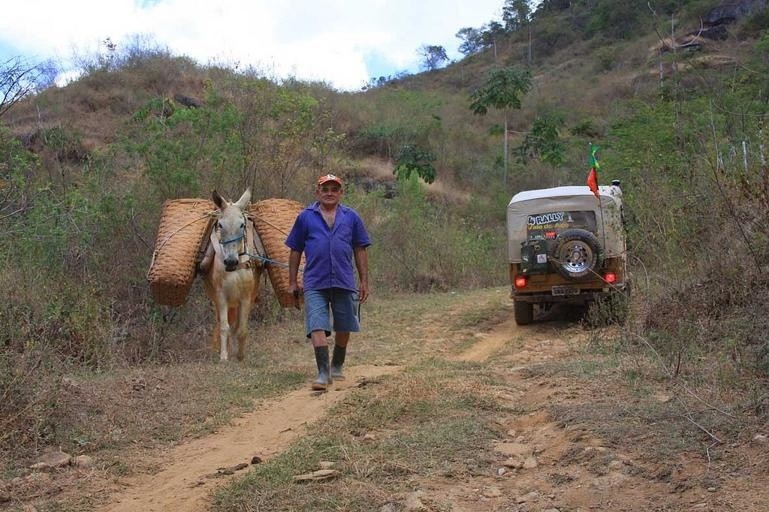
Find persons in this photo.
[285,173,372,391]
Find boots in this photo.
[312,345,332,389]
[331,344,346,381]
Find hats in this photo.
[316,174,343,188]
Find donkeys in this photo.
[202,184,266,363]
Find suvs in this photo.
[505,181,632,326]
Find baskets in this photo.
[248,198,307,308]
[145,196,217,306]
